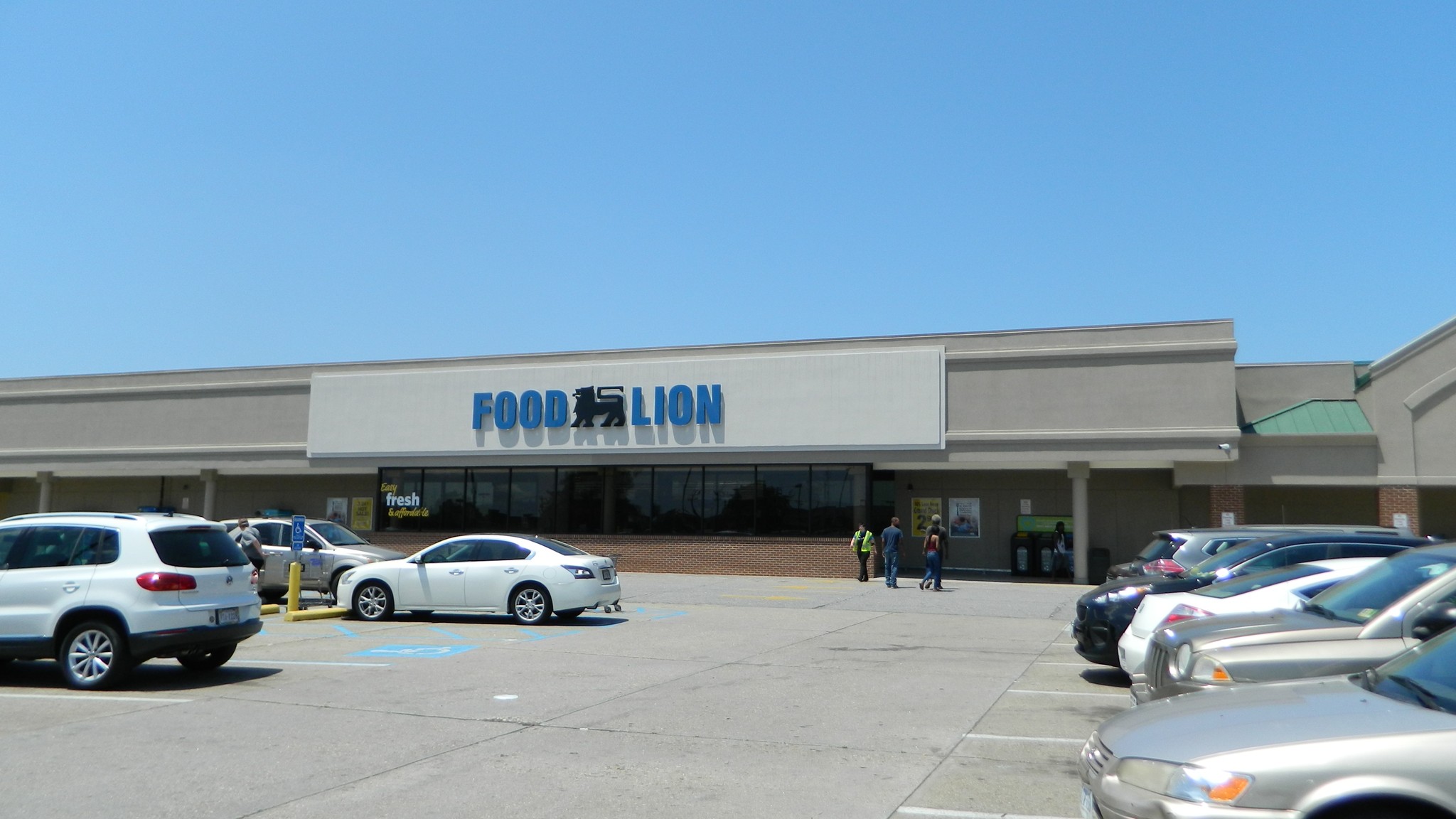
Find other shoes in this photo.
[938,586,943,589]
[891,585,898,588]
[919,582,923,590]
[933,588,940,591]
[887,585,891,587]
[924,578,932,588]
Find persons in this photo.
[920,525,942,592]
[924,514,949,589]
[1050,521,1074,583]
[850,522,877,582]
[238,518,265,578]
[880,518,906,588]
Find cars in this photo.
[218,518,410,599]
[337,532,621,625]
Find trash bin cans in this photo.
[1090,548,1110,585]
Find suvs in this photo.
[0,510,265,690]
[1070,523,1456,819]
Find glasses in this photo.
[858,526,864,528]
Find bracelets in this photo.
[882,552,884,553]
